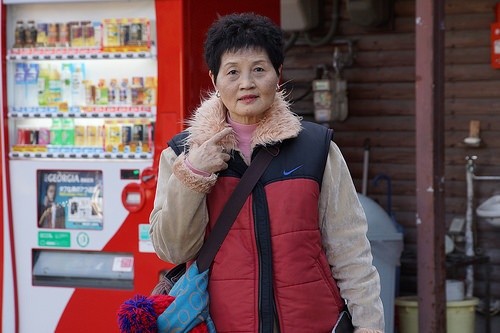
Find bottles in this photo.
[101,119,154,152]
[50,118,75,145]
[14,19,148,49]
[11,63,158,114]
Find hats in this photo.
[115,294,175,333]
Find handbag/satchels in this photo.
[157,263,218,333]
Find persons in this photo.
[149,12,387,333]
[38,183,64,229]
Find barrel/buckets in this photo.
[394,295,481,333]
